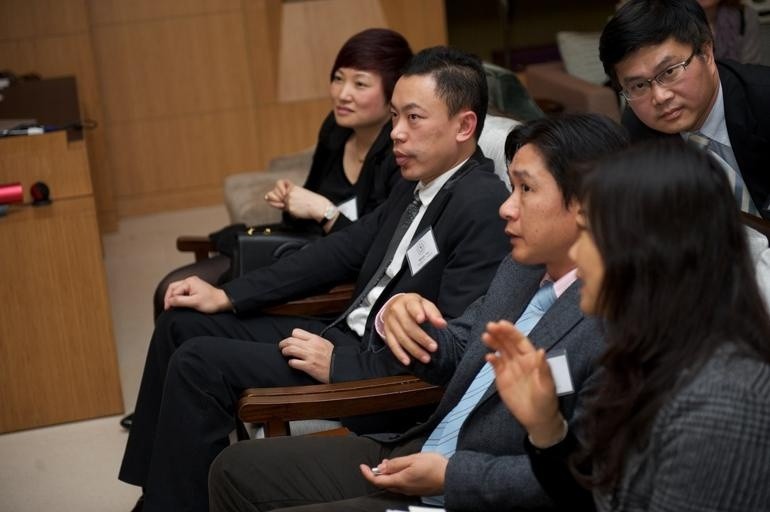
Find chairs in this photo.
[176,110,770,437]
[525,62,622,121]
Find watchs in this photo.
[317,202,336,225]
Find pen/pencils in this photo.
[372,466,380,472]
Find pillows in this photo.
[555,27,611,87]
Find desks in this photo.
[0,107,126,436]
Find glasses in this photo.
[618,52,696,100]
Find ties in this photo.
[683,131,762,219]
[317,191,422,337]
[418,276,559,510]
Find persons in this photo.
[206,111,639,512]
[597,1,769,227]
[115,44,514,512]
[118,26,418,431]
[480,135,769,512]
[696,1,765,67]
